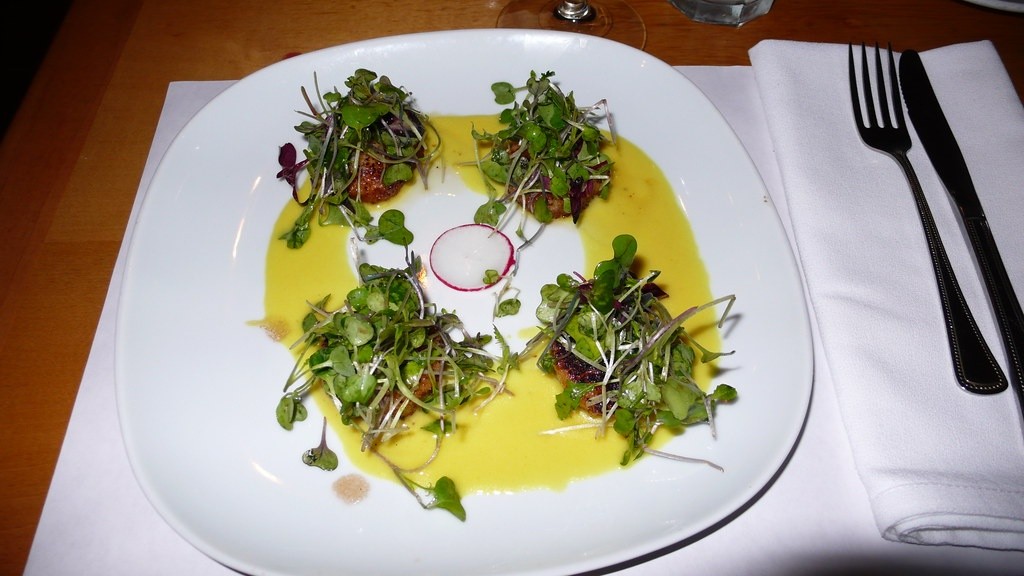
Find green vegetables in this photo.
[276,67,737,523]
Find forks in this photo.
[843,39,1010,395]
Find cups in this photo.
[669,0,773,28]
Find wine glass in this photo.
[496,0,647,52]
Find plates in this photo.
[112,28,816,575]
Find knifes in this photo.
[896,44,1024,413]
